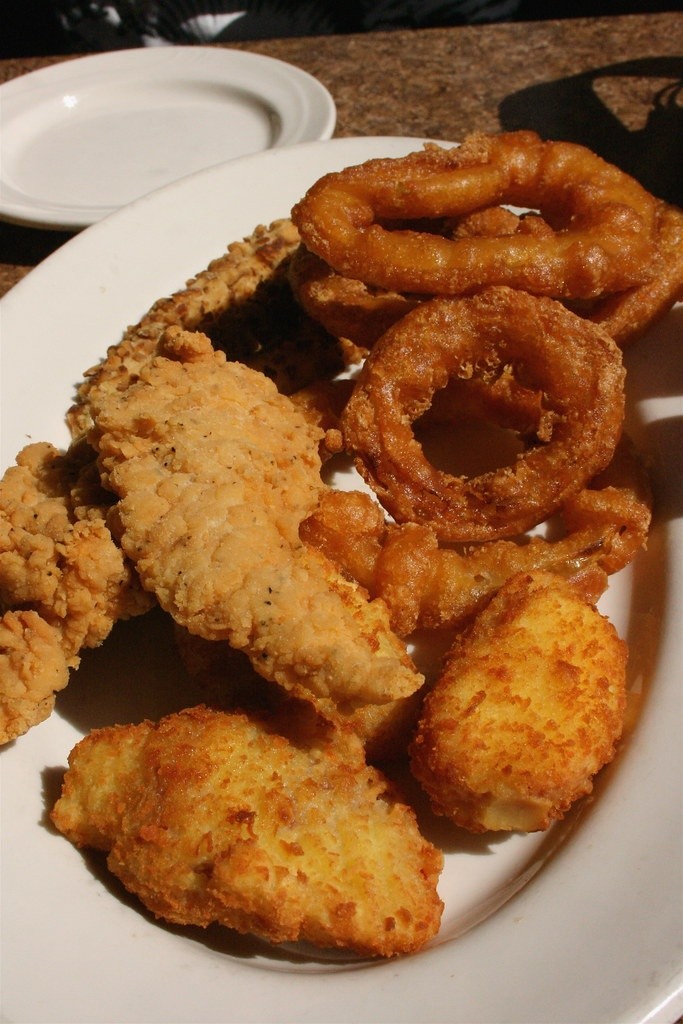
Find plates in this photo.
[0,45,336,232]
[0,134,683,1024]
